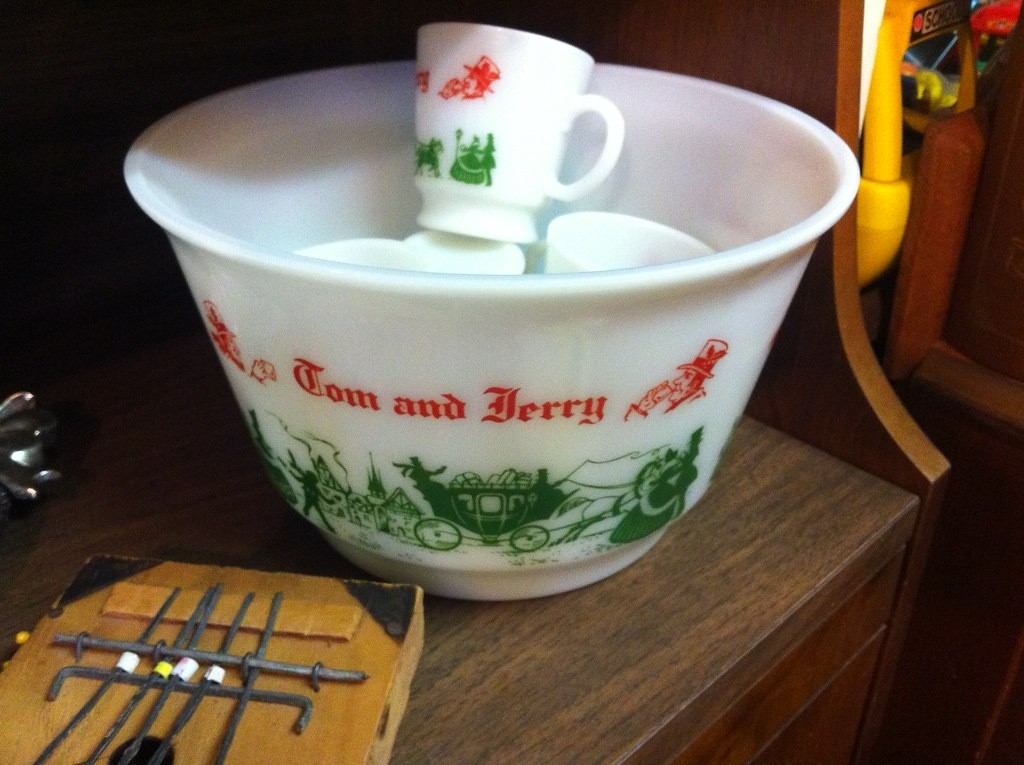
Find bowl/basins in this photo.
[124,59,862,601]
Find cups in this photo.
[302,22,719,280]
